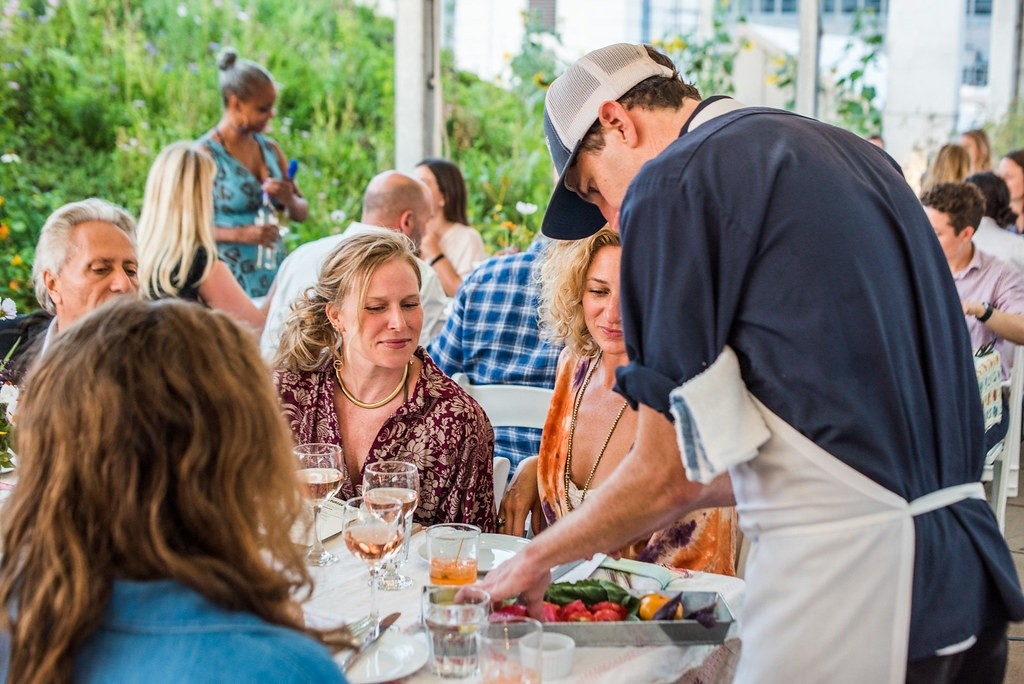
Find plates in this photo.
[334,629,430,684]
[486,590,737,648]
[417,533,533,575]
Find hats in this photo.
[541,42,684,242]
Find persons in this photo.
[454,41,1024,684]
[867,133,885,149]
[256,169,451,390]
[133,141,272,345]
[264,231,498,536]
[960,171,1024,274]
[921,143,972,192]
[411,158,487,302]
[190,48,309,301]
[960,129,992,172]
[998,148,1024,237]
[918,181,1024,450]
[0,197,142,445]
[0,295,351,683]
[497,229,736,578]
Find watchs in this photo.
[975,301,994,322]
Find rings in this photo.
[497,517,505,526]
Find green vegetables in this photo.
[544,579,641,621]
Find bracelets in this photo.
[429,253,444,267]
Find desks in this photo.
[0,445,747,684]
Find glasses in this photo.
[974,338,997,358]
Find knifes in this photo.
[341,612,401,674]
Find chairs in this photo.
[451,371,553,430]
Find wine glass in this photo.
[290,442,345,567]
[342,494,404,644]
[361,461,420,591]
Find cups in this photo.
[425,522,482,588]
[476,615,544,684]
[518,631,575,680]
[422,585,492,684]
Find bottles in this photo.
[254,179,280,271]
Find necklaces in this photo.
[565,350,629,514]
[335,357,407,409]
[214,125,231,156]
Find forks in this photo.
[346,615,374,638]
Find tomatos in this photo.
[640,595,683,620]
[495,600,626,622]
